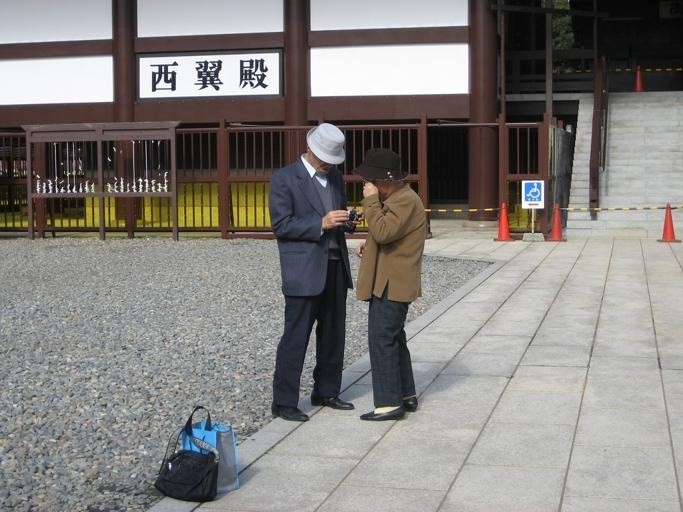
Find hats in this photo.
[350,148,408,182]
[306,122,345,164]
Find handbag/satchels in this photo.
[155,429,218,502]
[183,406,239,494]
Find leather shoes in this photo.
[272,406,309,421]
[311,397,354,409]
[404,397,418,412]
[360,405,404,420]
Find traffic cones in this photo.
[494,201,516,242]
[546,203,567,242]
[656,203,681,243]
[634,66,645,92]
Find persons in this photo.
[268,123,354,421]
[352,146,428,422]
